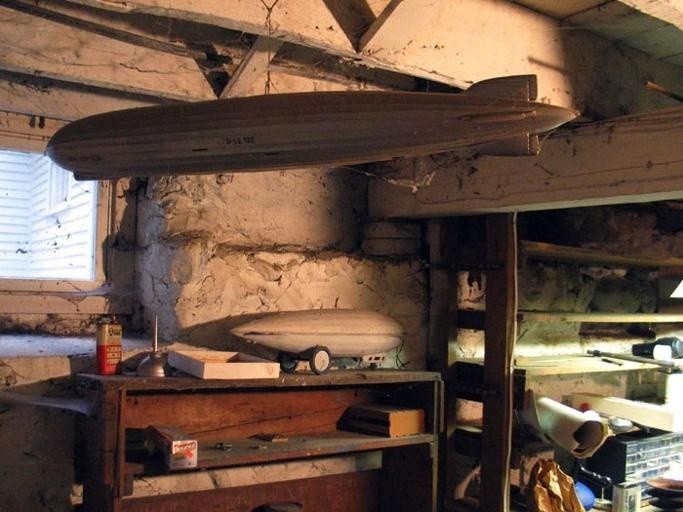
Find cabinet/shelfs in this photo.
[74,370,444,512]
[426,211,683,512]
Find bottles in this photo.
[581,402,612,434]
